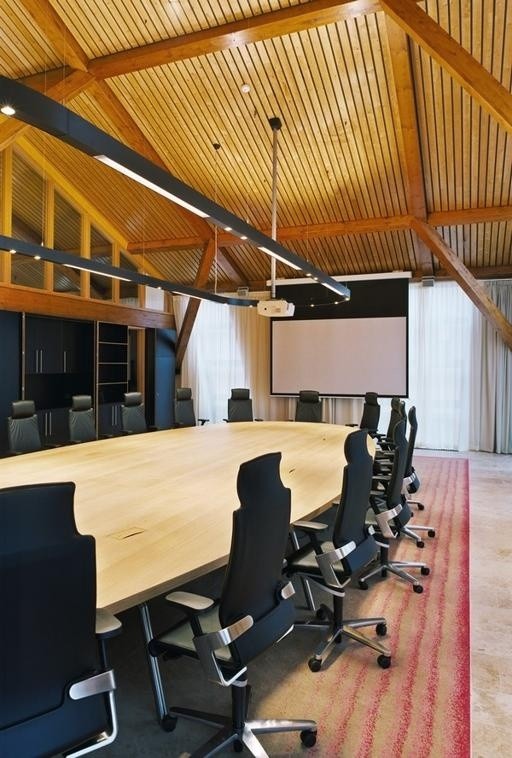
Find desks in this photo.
[2,420,376,724]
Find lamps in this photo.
[0,75,351,310]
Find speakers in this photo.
[237,289,248,297]
[422,279,435,288]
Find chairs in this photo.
[121,392,148,435]
[286,429,393,672]
[341,392,436,594]
[174,388,209,427]
[7,399,42,454]
[2,481,122,758]
[289,391,323,422]
[69,396,96,444]
[222,389,264,423]
[139,452,318,758]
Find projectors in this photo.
[257,299,295,317]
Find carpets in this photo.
[84,456,471,758]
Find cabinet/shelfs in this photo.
[92,319,128,438]
[60,319,92,373]
[21,316,63,374]
